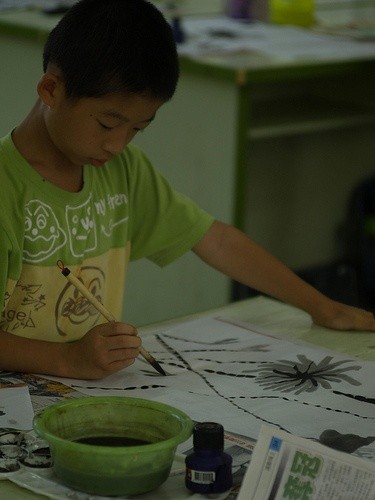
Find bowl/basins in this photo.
[32,395,193,497]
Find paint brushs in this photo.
[56,259,168,379]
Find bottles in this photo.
[186,421,232,495]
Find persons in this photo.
[0,2,374,380]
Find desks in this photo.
[0,295,375,500]
[1,1,375,324]
